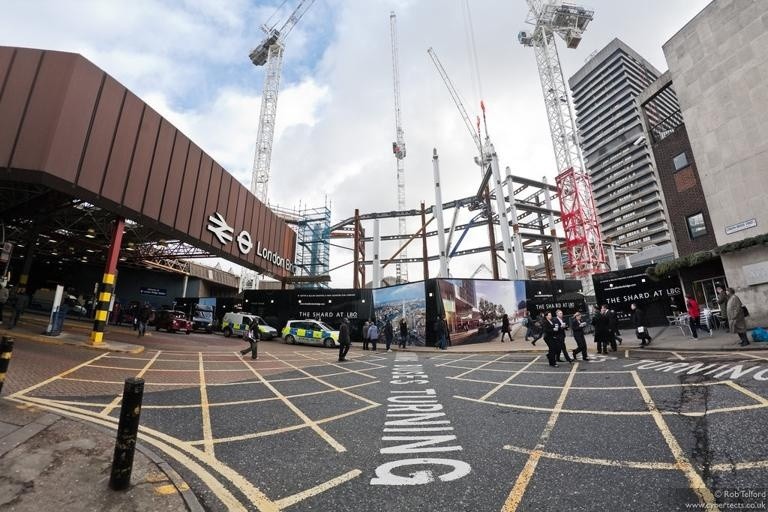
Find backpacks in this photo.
[522,319,528,326]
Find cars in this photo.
[491,322,497,326]
[280,318,341,349]
[478,323,495,333]
[84,299,97,312]
[155,309,192,336]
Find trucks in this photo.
[120,301,156,325]
[32,288,87,317]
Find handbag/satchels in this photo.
[243,331,249,341]
[248,329,259,341]
[742,306,749,317]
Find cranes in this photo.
[385,11,410,283]
[426,42,493,175]
[246,1,318,209]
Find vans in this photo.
[190,303,217,334]
[221,311,278,344]
